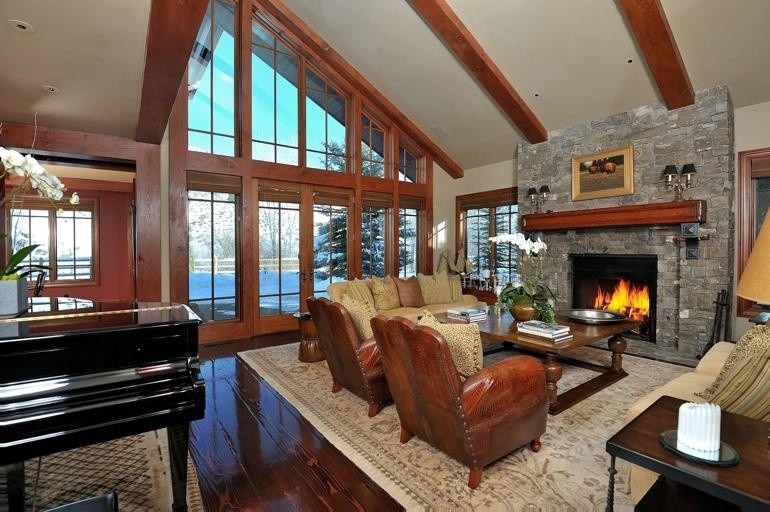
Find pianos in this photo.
[0,296,206,512]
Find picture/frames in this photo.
[571,144,634,202]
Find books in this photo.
[516,320,573,342]
[447,309,487,323]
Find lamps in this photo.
[737,203,770,305]
[662,164,696,201]
[528,186,550,214]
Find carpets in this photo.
[1,427,207,512]
[236,339,696,510]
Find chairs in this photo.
[308,294,394,417]
[370,313,549,487]
[33,271,47,296]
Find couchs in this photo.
[625,326,769,502]
[328,275,497,320]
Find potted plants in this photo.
[496,279,556,323]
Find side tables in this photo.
[604,396,770,511]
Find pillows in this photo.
[694,324,769,421]
[371,273,463,310]
[345,277,374,307]
[417,310,484,380]
[342,295,373,340]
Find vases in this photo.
[0,316,30,340]
[1,276,28,315]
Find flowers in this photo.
[489,232,548,276]
[1,147,78,280]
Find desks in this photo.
[748,311,770,325]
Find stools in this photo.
[289,307,325,364]
[42,491,120,512]
